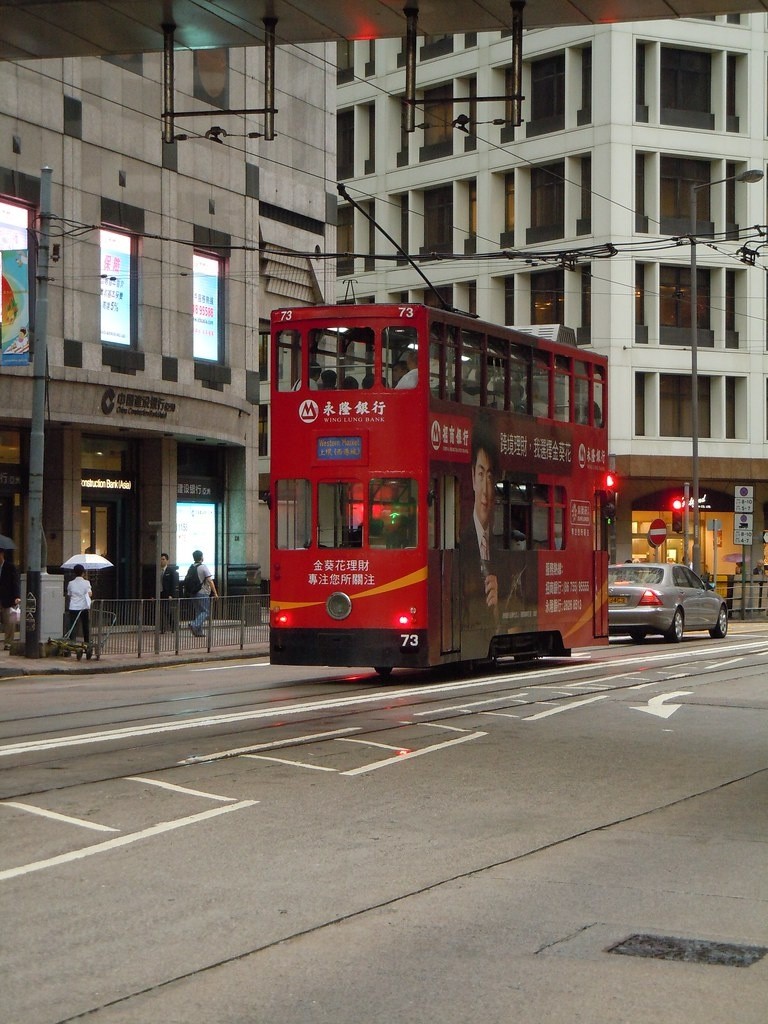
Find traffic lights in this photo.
[672,495,683,533]
[606,472,615,526]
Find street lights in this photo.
[690,168,763,574]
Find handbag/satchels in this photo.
[9,603,21,623]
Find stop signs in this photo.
[648,518,667,546]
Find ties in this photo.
[479,531,490,573]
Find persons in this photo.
[291,362,322,393]
[0,548,21,650]
[394,350,419,389]
[753,559,764,576]
[381,377,390,388]
[682,556,694,572]
[734,561,743,574]
[510,506,551,551]
[342,377,360,390]
[160,553,181,634]
[317,370,338,391]
[393,361,409,382]
[188,550,220,638]
[624,560,634,564]
[66,564,93,643]
[347,512,415,549]
[448,413,506,662]
[430,354,605,430]
[362,373,374,389]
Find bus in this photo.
[270,304,613,681]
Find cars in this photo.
[608,562,729,643]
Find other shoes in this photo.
[189,622,198,637]
[196,632,207,637]
[3,645,11,650]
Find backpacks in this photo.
[184,563,205,596]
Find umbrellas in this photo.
[60,554,114,571]
[0,535,17,550]
[721,553,751,563]
